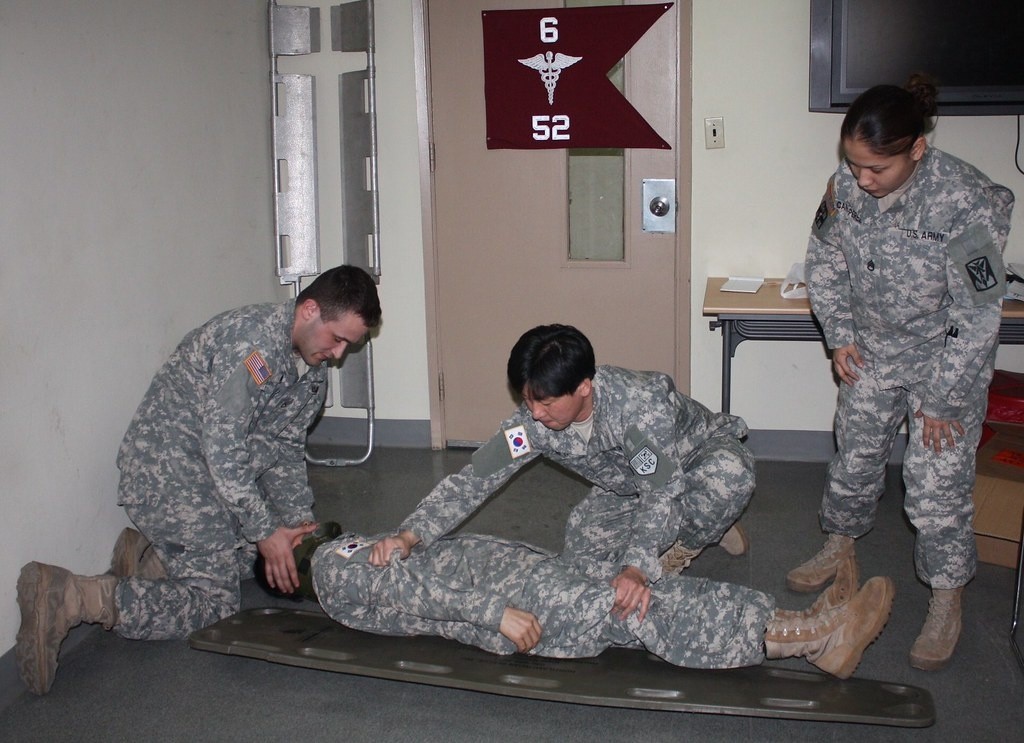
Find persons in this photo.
[367,322,757,626]
[785,73,1016,673]
[18,264,383,697]
[254,522,896,680]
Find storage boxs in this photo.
[971,422,1024,570]
[977,368,1024,449]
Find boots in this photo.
[911,587,962,668]
[16,562,118,694]
[767,559,893,678]
[113,528,166,577]
[786,535,853,591]
[719,520,748,555]
[660,542,702,574]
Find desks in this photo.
[703,277,1024,414]
